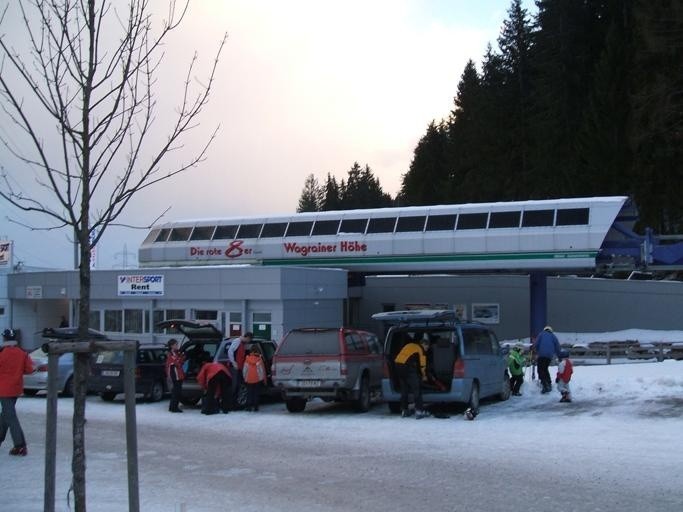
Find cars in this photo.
[89,341,174,404]
[23,339,83,399]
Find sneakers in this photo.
[169,402,262,415]
[8,445,28,456]
[400,408,431,419]
[512,381,572,404]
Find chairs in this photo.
[432,338,456,382]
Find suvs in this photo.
[269,327,385,413]
[158,320,278,404]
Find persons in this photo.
[0,327,34,456]
[394,338,432,418]
[243,344,268,411]
[226,332,253,410]
[556,346,572,403]
[59,316,68,328]
[166,339,185,412]
[510,343,526,397]
[533,326,561,393]
[197,360,232,414]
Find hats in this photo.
[513,341,526,350]
[544,325,554,333]
[1,328,16,338]
[557,349,570,358]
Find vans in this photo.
[371,307,512,417]
